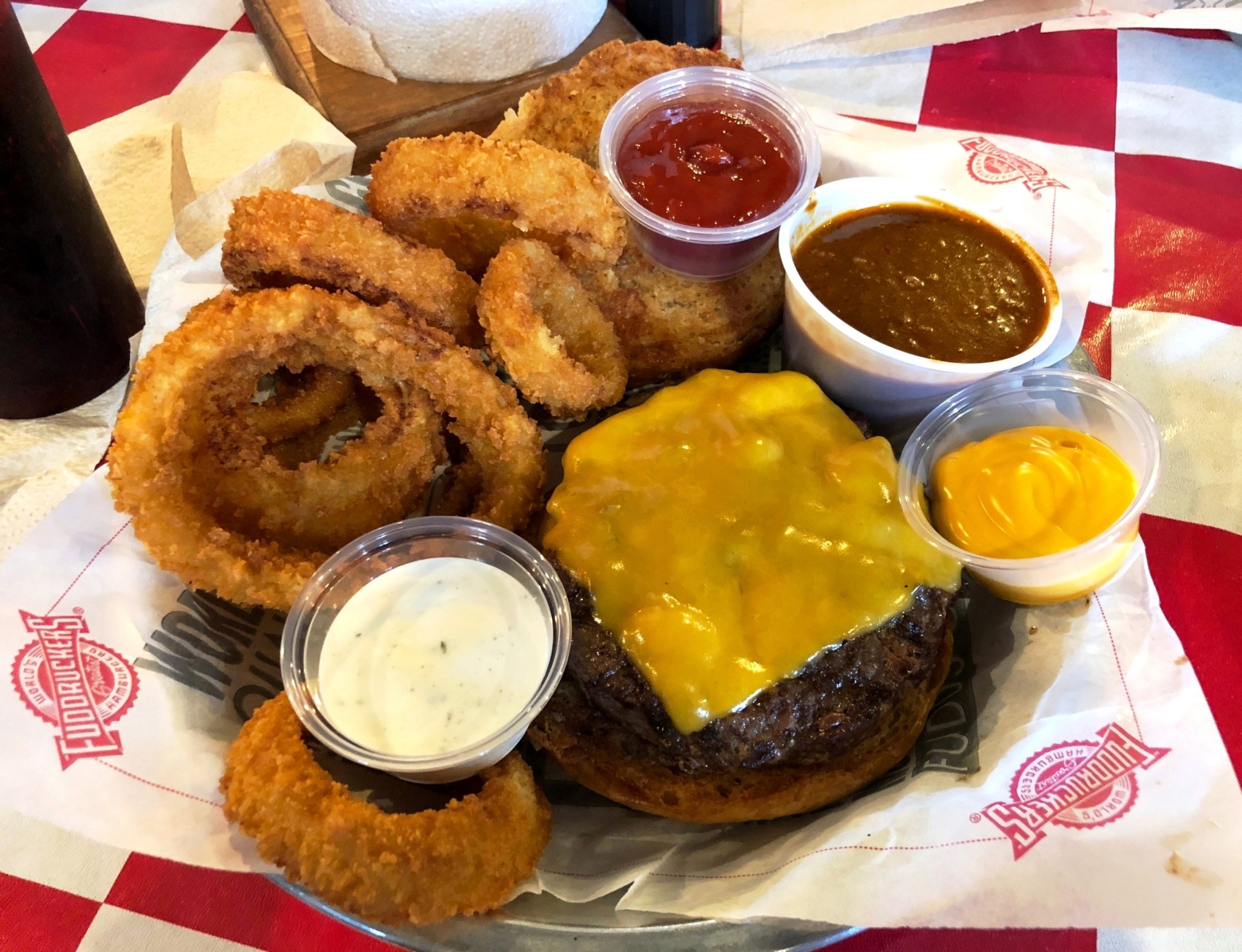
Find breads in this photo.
[481,38,820,369]
[299,0,609,83]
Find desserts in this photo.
[500,378,964,819]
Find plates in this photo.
[260,870,864,952]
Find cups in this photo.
[280,514,573,785]
[597,66,821,284]
[778,174,1064,424]
[898,367,1163,606]
